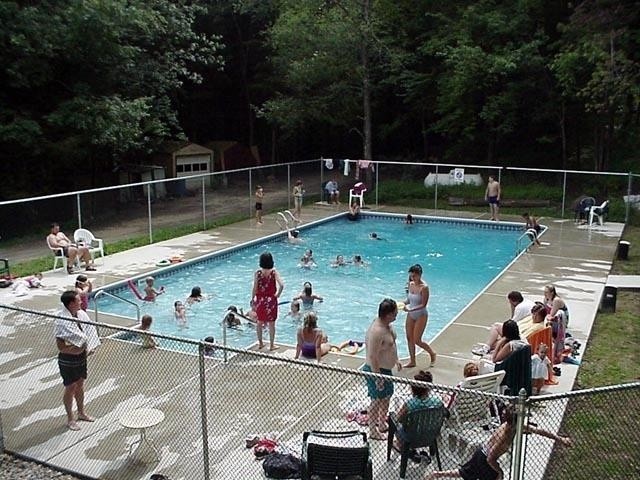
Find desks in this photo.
[117,407,167,465]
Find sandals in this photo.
[85,265,96,271]
[67,264,74,274]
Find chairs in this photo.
[478,343,532,396]
[299,428,376,480]
[525,325,554,383]
[321,180,340,204]
[584,199,611,228]
[45,235,81,275]
[439,370,509,468]
[0,257,13,286]
[386,403,446,478]
[73,228,106,266]
[348,181,368,209]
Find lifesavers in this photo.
[156,254,184,266]
[331,340,367,355]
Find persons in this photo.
[363,299,401,440]
[144,277,164,301]
[298,249,364,266]
[286,226,303,243]
[370,232,381,240]
[399,264,437,368]
[519,212,541,245]
[484,176,500,221]
[203,337,215,357]
[325,176,341,204]
[11,272,47,290]
[295,312,331,363]
[255,184,264,225]
[288,280,322,317]
[252,252,283,350]
[403,215,414,224]
[580,191,609,224]
[48,223,96,274]
[291,179,303,223]
[53,275,95,431]
[349,202,360,219]
[139,314,158,348]
[226,299,264,328]
[388,285,568,480]
[174,286,204,320]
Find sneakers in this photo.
[471,342,490,356]
